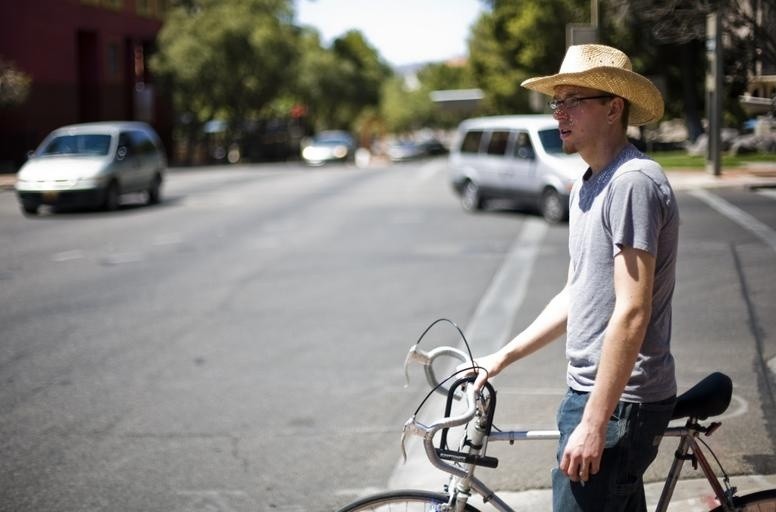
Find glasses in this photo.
[548,94,614,110]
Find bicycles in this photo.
[331,317,776,510]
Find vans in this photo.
[447,114,590,223]
[15,122,167,217]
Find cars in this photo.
[356,130,448,167]
[300,132,358,167]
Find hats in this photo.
[519,43,665,127]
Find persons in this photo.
[457,84,679,511]
